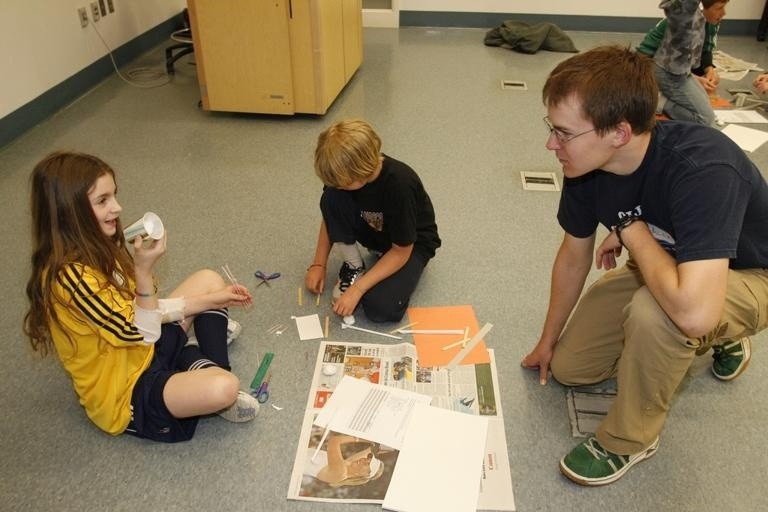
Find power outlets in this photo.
[78,7,88,28]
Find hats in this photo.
[222,320,243,344]
[210,389,261,425]
[330,261,365,314]
[556,429,660,489]
[708,336,752,383]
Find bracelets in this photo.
[135,284,157,296]
[351,436,359,443]
[307,265,326,271]
[616,216,648,251]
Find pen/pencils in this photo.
[324,316,329,338]
[462,326,469,347]
[298,288,302,308]
[316,292,320,307]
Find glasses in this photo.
[543,115,597,144]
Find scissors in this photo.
[254,271,280,288]
[251,373,272,403]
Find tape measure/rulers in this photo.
[251,352,275,389]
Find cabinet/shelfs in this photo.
[183,0,365,123]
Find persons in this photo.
[521,45,768,487]
[635,0,730,91]
[22,151,261,443]
[304,120,441,323]
[301,435,385,487]
[751,72,768,93]
[651,0,716,126]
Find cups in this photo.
[734,93,747,108]
[124,212,163,244]
